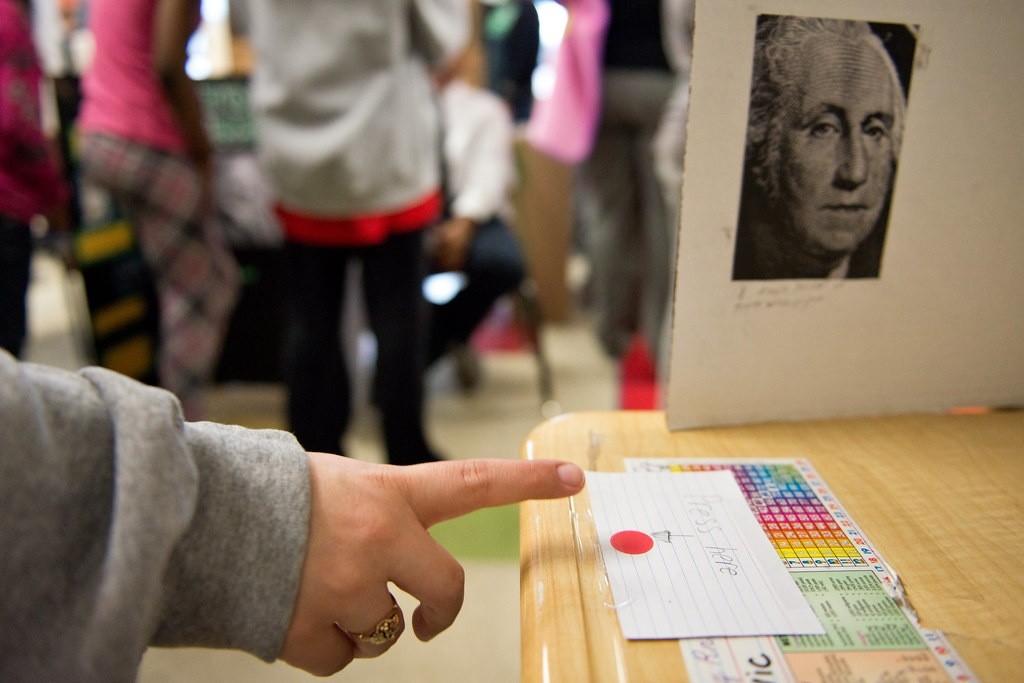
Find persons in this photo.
[356,25,528,420]
[0,340,583,683]
[733,14,903,283]
[72,1,248,422]
[0,0,76,370]
[245,1,458,468]
[534,0,694,416]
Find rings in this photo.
[350,594,402,648]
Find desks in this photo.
[521,414,1024,683]
